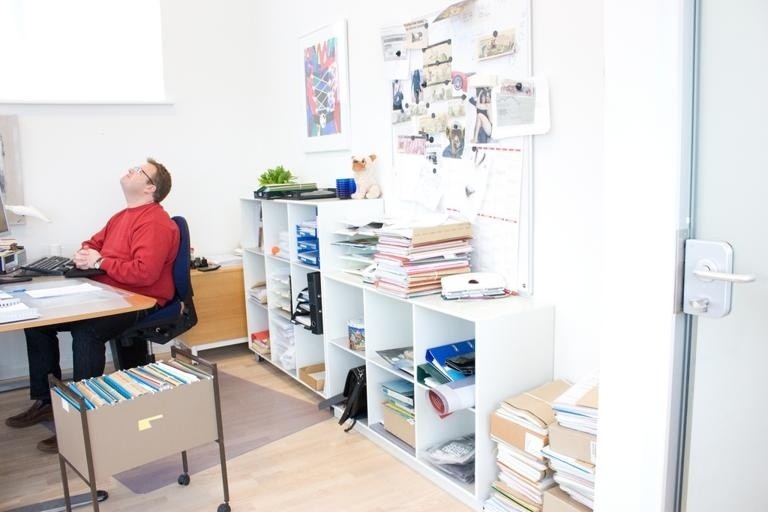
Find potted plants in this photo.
[257,163,297,198]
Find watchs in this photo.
[93,258,102,270]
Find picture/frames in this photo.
[0,112,29,227]
[294,16,354,155]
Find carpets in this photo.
[15,361,338,497]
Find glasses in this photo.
[133,166,155,186]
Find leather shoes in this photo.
[6,400,54,428]
[37,433,60,454]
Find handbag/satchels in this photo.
[338,365,367,434]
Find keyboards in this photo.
[23,256,75,273]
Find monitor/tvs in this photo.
[0,194,12,238]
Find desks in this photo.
[0,260,183,510]
[175,251,249,364]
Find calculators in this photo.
[437,439,475,465]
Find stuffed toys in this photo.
[350,154,382,200]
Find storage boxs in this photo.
[48,357,220,486]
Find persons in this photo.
[472,89,492,143]
[6,158,181,454]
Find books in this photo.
[377,336,474,424]
[372,219,510,300]
[0,288,35,325]
[488,378,595,511]
[55,359,203,412]
[251,221,313,371]
[254,180,322,200]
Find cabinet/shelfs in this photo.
[239,195,556,511]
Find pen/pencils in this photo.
[7,289,26,293]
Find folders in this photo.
[307,272,323,334]
